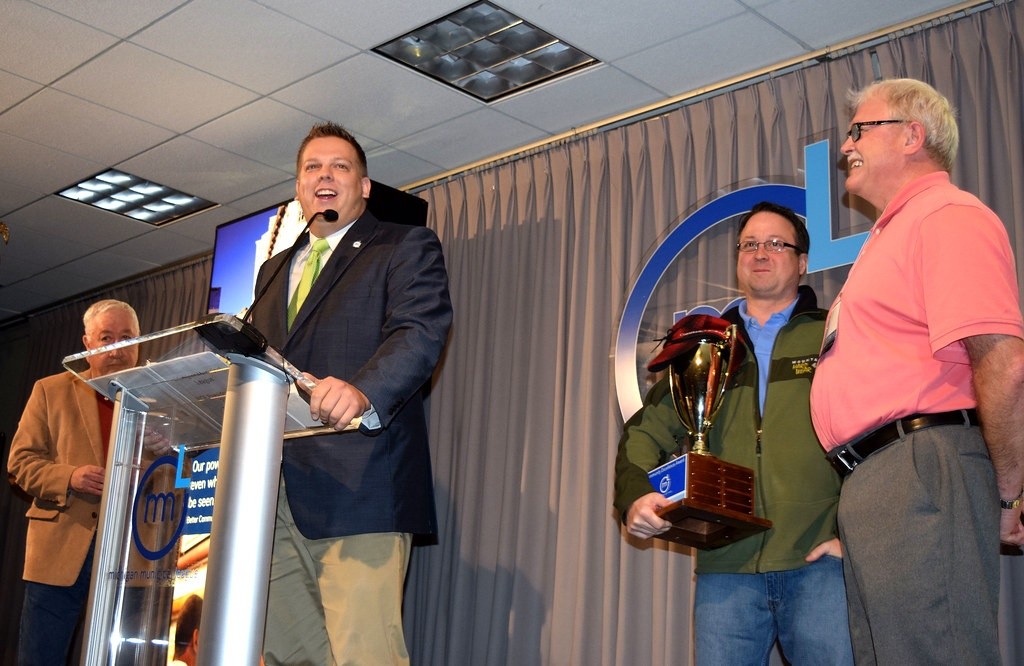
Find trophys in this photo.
[646,314,772,550]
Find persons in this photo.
[806,78,1024,664]
[136,122,453,666]
[611,201,854,666]
[7,300,153,664]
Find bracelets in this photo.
[1000,489,1024,509]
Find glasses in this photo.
[737,240,802,253]
[847,120,912,143]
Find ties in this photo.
[287,237,330,332]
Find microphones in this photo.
[194,209,339,353]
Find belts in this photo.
[833,410,980,475]
